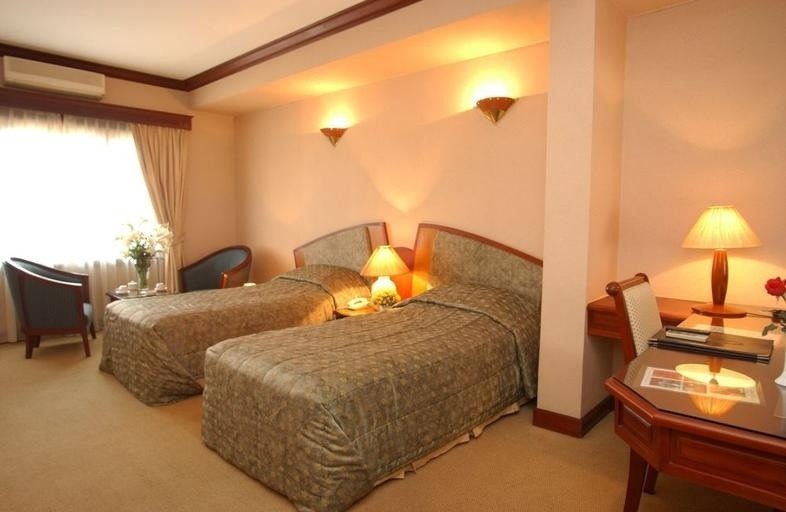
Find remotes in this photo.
[665,326,711,334]
[666,330,709,342]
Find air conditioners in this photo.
[1,53,106,102]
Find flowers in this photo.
[761,275,786,337]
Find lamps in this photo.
[475,95,516,126]
[319,127,350,147]
[674,353,755,419]
[677,203,764,320]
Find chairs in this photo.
[607,270,666,357]
[1,251,97,360]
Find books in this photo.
[648,324,775,363]
[664,325,711,344]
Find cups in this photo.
[117,281,168,296]
